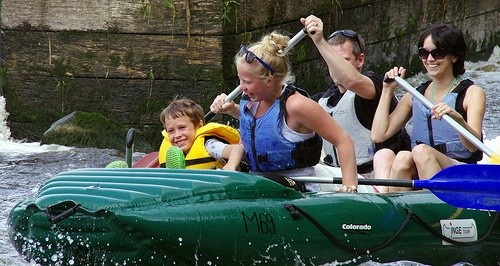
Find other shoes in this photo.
[105,161,128,168]
[166,146,186,170]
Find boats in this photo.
[7,166,500,266]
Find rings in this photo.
[316,23,318,26]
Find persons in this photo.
[106,99,245,171]
[209,32,373,195]
[301,14,412,194]
[370,24,486,192]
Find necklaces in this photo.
[431,78,456,105]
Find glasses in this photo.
[328,30,362,53]
[418,47,449,59]
[238,47,273,75]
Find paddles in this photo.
[133,26,315,168]
[289,165,500,211]
[383,73,500,164]
[126,128,133,168]
